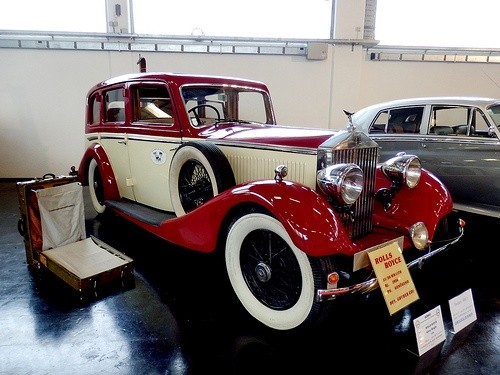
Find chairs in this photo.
[108,108,125,121]
[384,114,474,135]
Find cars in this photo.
[70,54,466,332]
[342,96,500,219]
